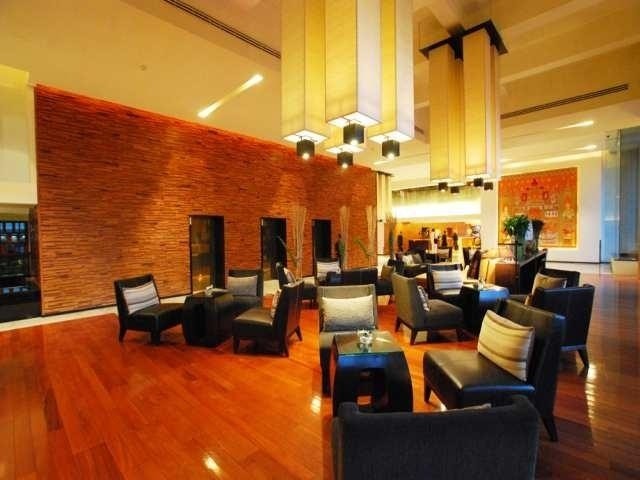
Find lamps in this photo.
[419,20,508,195]
[281,1,415,170]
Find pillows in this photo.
[122,280,159,315]
[270,290,280,319]
[228,275,258,296]
[284,268,295,283]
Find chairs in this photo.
[228,270,264,310]
[276,262,317,309]
[232,280,304,357]
[114,273,185,346]
